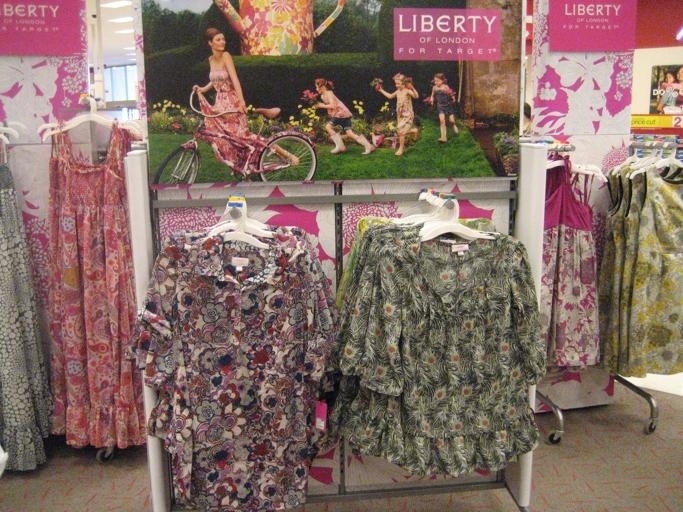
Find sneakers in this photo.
[395,148,404,155]
[412,129,419,141]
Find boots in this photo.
[331,132,346,154]
[452,123,458,133]
[356,134,376,154]
[439,126,447,143]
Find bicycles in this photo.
[153,87,318,185]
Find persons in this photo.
[674,58,682,116]
[193,27,299,168]
[427,73,456,143]
[655,70,676,115]
[312,78,376,156]
[373,74,418,157]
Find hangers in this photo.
[546,142,608,185]
[36,94,145,144]
[393,189,500,243]
[205,193,275,251]
[1,117,29,149]
[607,136,682,179]
